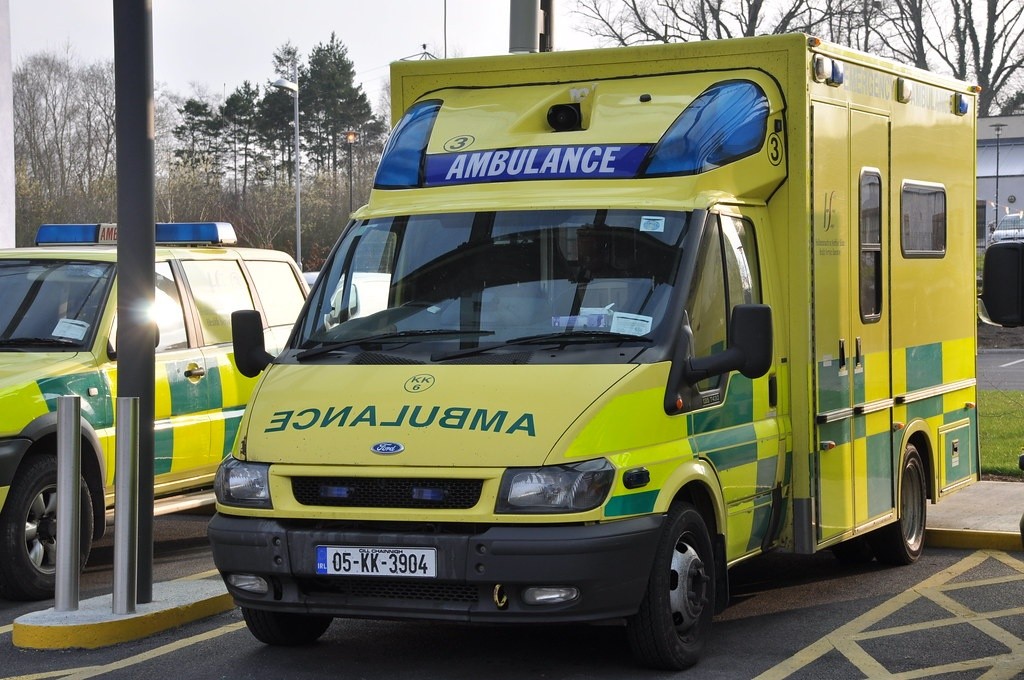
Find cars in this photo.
[990,213,1024,243]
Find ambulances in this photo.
[207,32,982,673]
[1,222,312,601]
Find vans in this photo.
[302,272,392,331]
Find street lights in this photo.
[272,78,303,270]
[989,123,1008,227]
[340,130,361,212]
